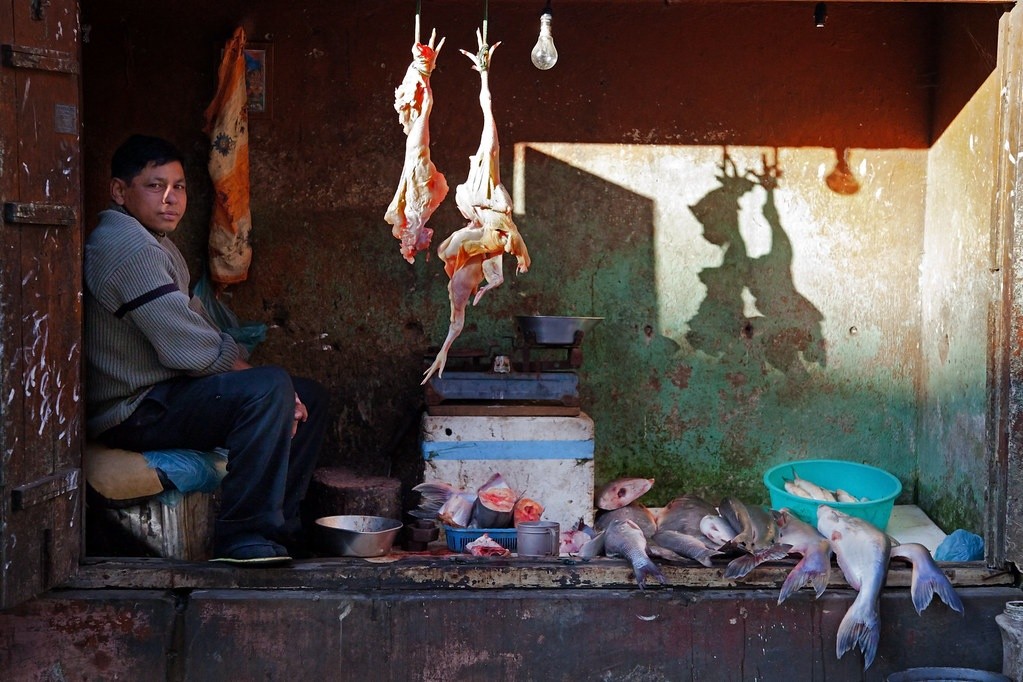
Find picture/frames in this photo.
[215,42,275,121]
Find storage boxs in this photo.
[420,414,596,551]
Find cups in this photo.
[494,356,510,373]
[517,519,561,557]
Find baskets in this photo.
[443,524,517,553]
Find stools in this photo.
[85,446,229,562]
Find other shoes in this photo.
[207,539,293,563]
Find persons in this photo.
[83,135,339,568]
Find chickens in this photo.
[380,41,450,267]
[417,24,533,389]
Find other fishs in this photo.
[404,466,963,672]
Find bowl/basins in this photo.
[315,515,403,558]
[762,459,902,533]
[515,315,607,344]
